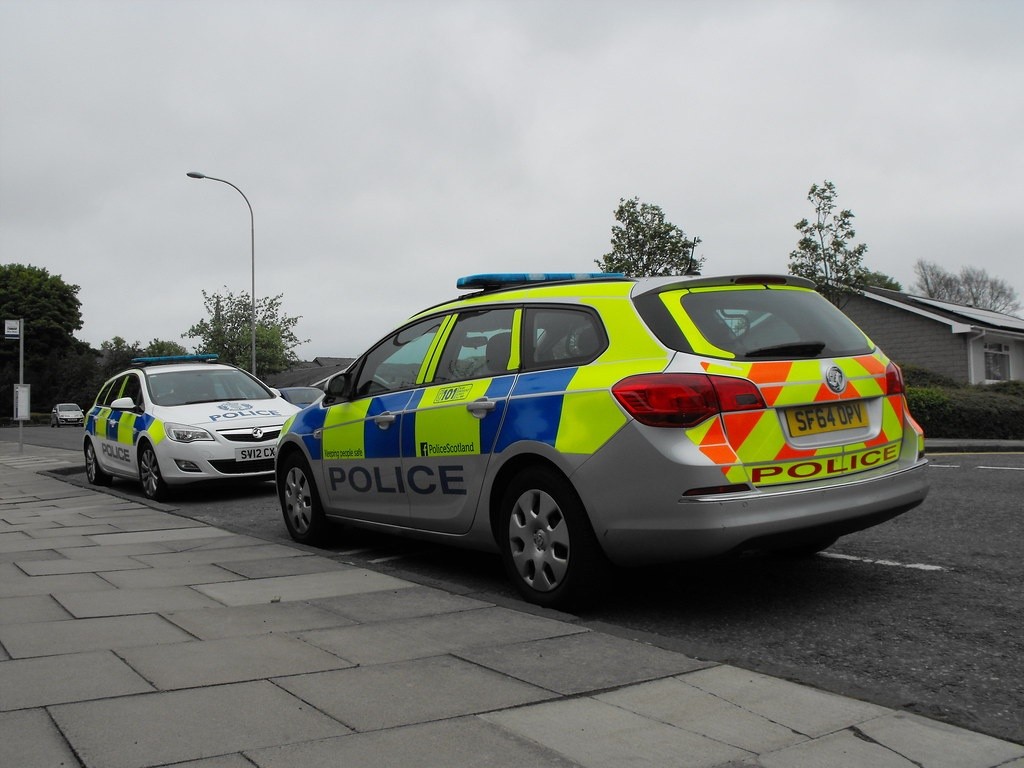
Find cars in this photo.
[82,355,306,502]
[49,403,86,427]
[279,387,324,411]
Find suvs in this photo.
[272,270,934,607]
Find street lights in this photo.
[185,171,258,380]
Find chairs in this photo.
[189,376,216,401]
[486,332,511,371]
[152,377,180,402]
[576,328,598,354]
